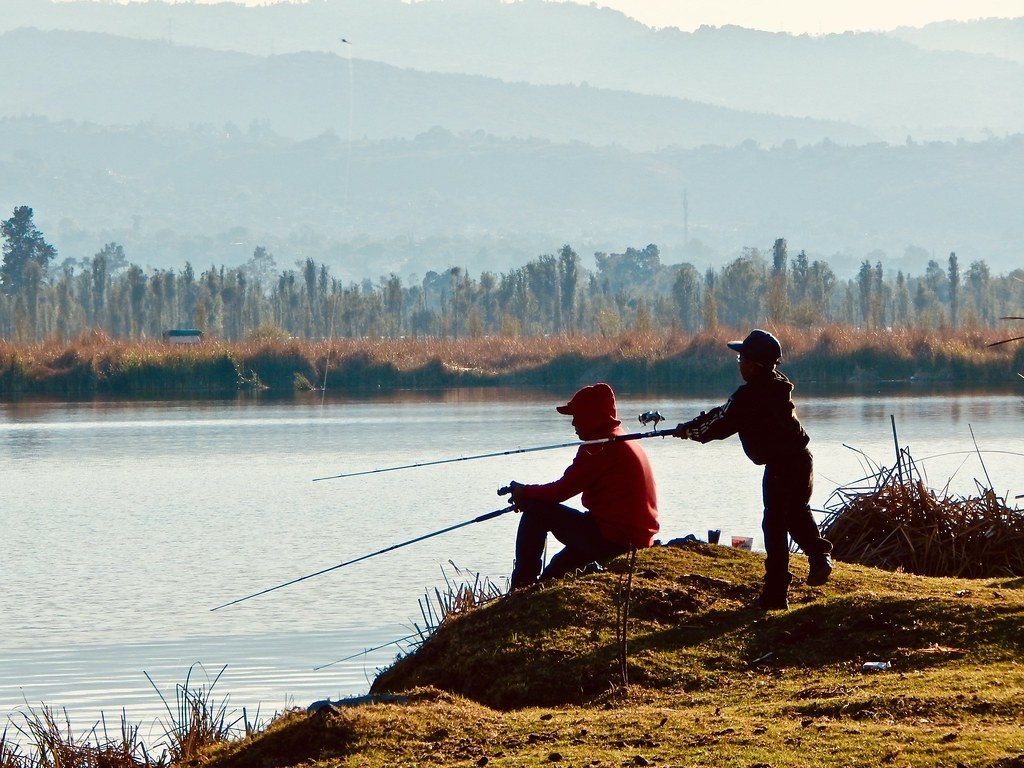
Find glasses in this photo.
[736,354,756,366]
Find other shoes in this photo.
[806,553,832,586]
[750,596,788,610]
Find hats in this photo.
[726,329,782,364]
[557,406,573,415]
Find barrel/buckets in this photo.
[732,535,754,552]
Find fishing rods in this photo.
[311,413,680,482]
[208,485,521,613]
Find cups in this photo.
[707,530,721,544]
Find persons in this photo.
[668,328,832,609]
[508,383,661,586]
